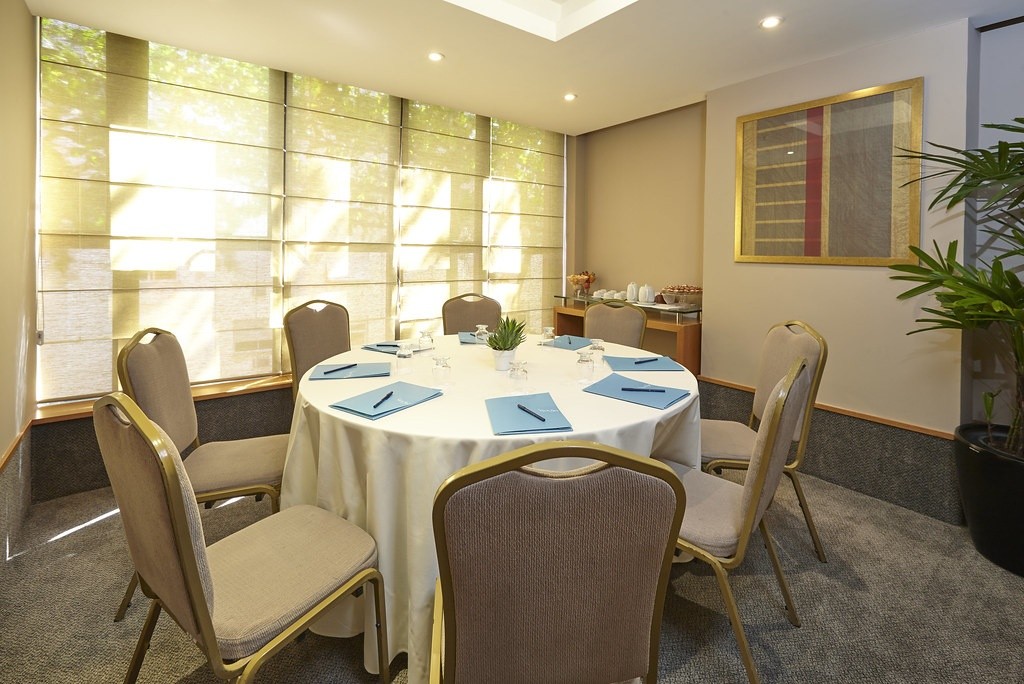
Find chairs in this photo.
[95,293,827,683]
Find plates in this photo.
[624,301,656,305]
[591,296,613,300]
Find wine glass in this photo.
[663,291,685,307]
[509,360,527,382]
[475,324,490,343]
[543,327,555,342]
[576,339,605,378]
[396,330,451,377]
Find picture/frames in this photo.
[734,76,924,266]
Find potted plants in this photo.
[486,313,527,370]
[889,117,1024,577]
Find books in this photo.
[362,341,401,353]
[458,331,490,343]
[605,355,684,371]
[327,382,444,421]
[309,363,390,379]
[582,372,689,409]
[540,335,591,351]
[485,393,574,435]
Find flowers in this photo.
[567,271,594,288]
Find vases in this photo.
[573,283,590,298]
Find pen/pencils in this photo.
[518,404,545,422]
[470,333,482,340]
[374,391,393,408]
[635,359,658,364]
[622,387,666,392]
[377,344,398,347]
[324,363,357,374]
[568,337,571,344]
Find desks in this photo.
[277,333,701,684]
[553,293,702,378]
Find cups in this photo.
[593,289,627,300]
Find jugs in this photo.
[639,284,655,303]
[627,281,638,301]
